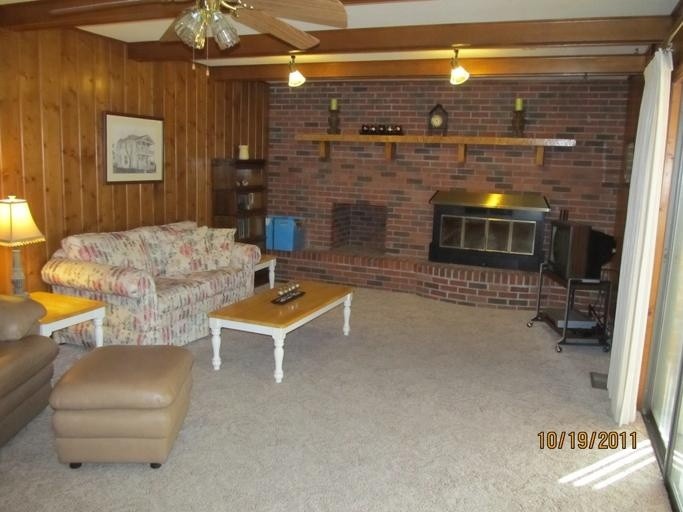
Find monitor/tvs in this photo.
[550,220,616,283]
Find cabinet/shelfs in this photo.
[524,259,613,356]
[208,154,269,289]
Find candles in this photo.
[513,96,522,114]
[328,97,338,111]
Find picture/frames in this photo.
[100,108,164,186]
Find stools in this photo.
[46,342,196,473]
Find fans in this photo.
[45,0,352,52]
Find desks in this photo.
[21,288,108,351]
[253,253,277,290]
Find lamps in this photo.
[443,45,471,88]
[284,55,305,90]
[171,1,241,80]
[0,193,46,297]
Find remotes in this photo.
[278,283,300,294]
[272,291,304,303]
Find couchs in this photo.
[0,291,61,452]
[37,217,263,352]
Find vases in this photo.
[237,145,249,161]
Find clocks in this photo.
[425,103,449,137]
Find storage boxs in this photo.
[262,213,308,256]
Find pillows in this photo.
[56,224,239,284]
